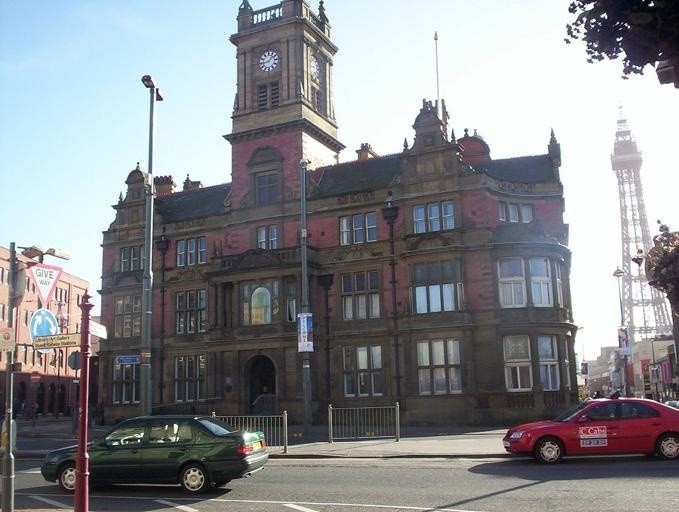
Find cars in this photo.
[501,390,679,465]
[39,412,271,496]
[664,400,679,410]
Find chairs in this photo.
[150,424,178,442]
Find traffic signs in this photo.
[115,354,141,365]
[32,332,81,349]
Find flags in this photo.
[581,362,588,375]
[617,326,678,401]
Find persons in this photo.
[584,393,593,400]
[608,389,623,398]
[593,391,604,399]
[32,400,40,420]
[20,399,27,417]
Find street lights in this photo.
[293,157,316,428]
[139,71,165,417]
[649,335,661,401]
[613,265,631,399]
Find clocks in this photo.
[258,49,279,72]
[310,55,321,79]
